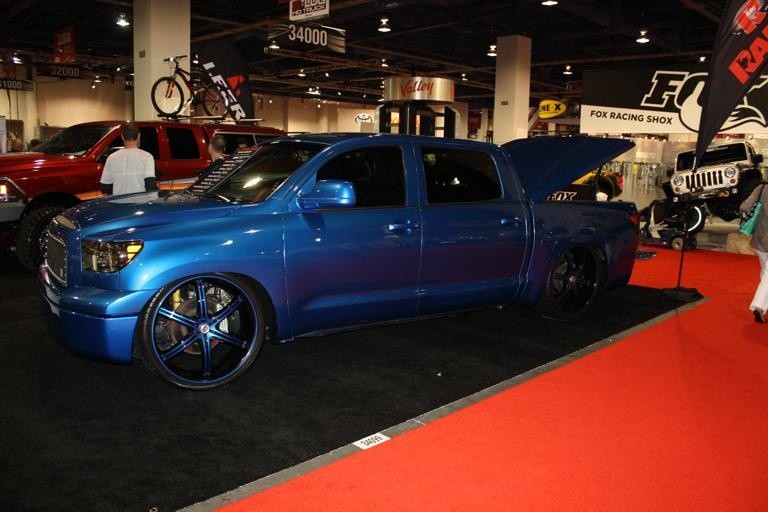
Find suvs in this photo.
[670,141,763,199]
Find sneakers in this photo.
[753,307,765,325]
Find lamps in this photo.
[114,0,129,28]
[378,15,391,33]
[487,44,497,56]
[636,30,650,45]
[563,65,572,75]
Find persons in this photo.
[6,139,26,152]
[99,122,157,196]
[207,136,231,163]
[738,182,768,322]
[29,139,40,150]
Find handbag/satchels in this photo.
[738,184,767,236]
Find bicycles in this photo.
[151,55,231,119]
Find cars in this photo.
[1,120,289,275]
[38,131,640,390]
